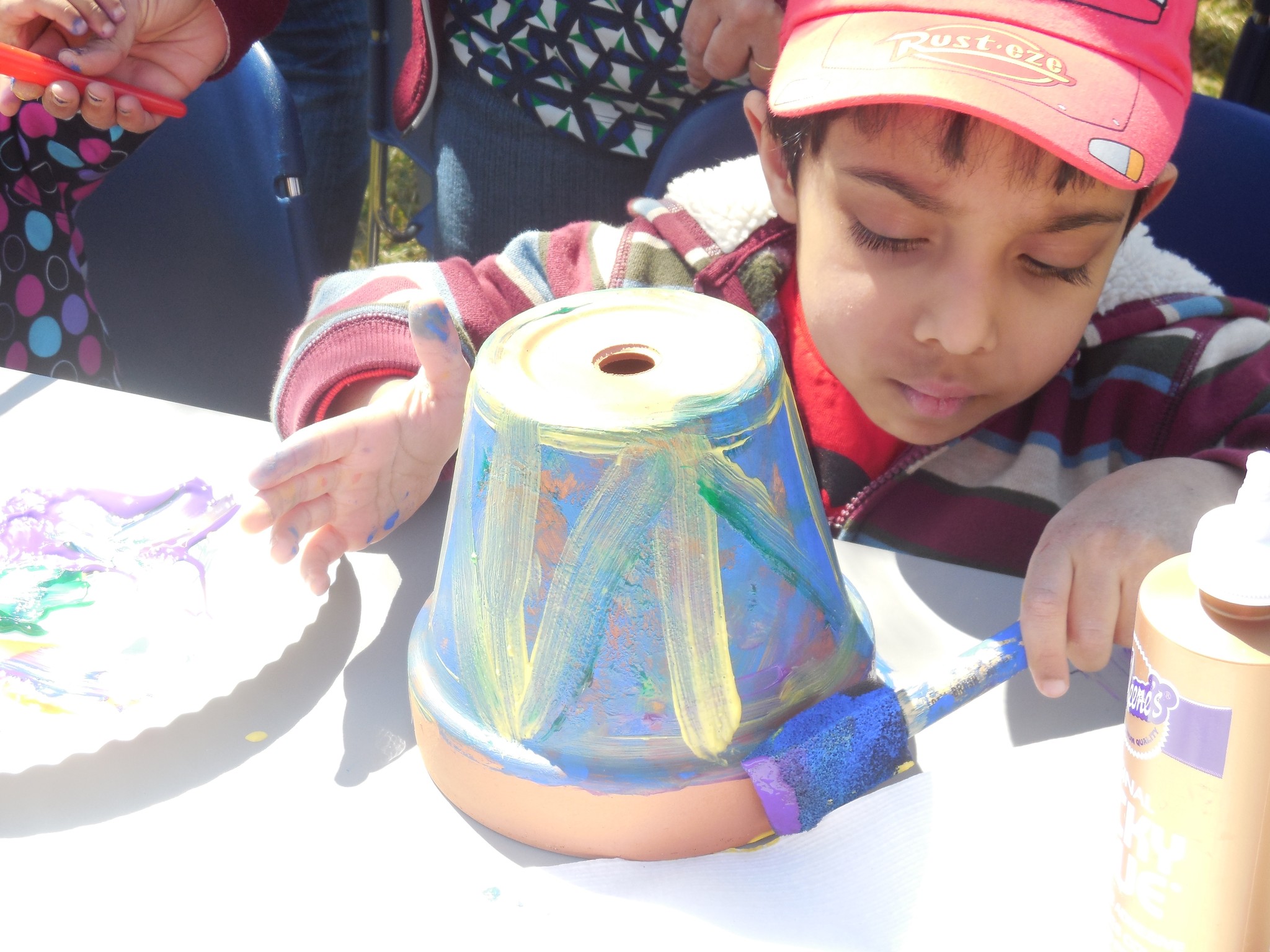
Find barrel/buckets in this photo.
[410,289,912,859]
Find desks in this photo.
[0,356,1139,951]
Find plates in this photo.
[0,410,343,776]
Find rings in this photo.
[749,54,777,73]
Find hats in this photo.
[764,1,1198,190]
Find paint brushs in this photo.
[750,620,1028,839]
[1,39,187,121]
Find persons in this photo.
[0,0,161,395]
[234,0,1270,704]
[6,0,791,272]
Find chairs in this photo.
[76,37,309,423]
[644,84,1269,316]
